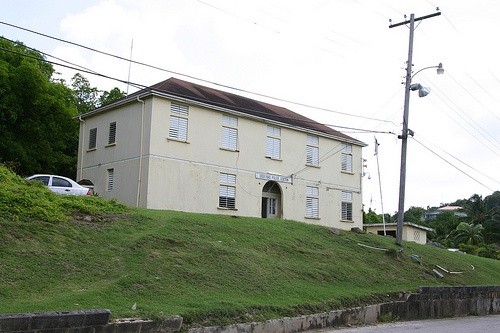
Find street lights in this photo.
[396,14,444,246]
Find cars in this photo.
[23,175,92,197]
